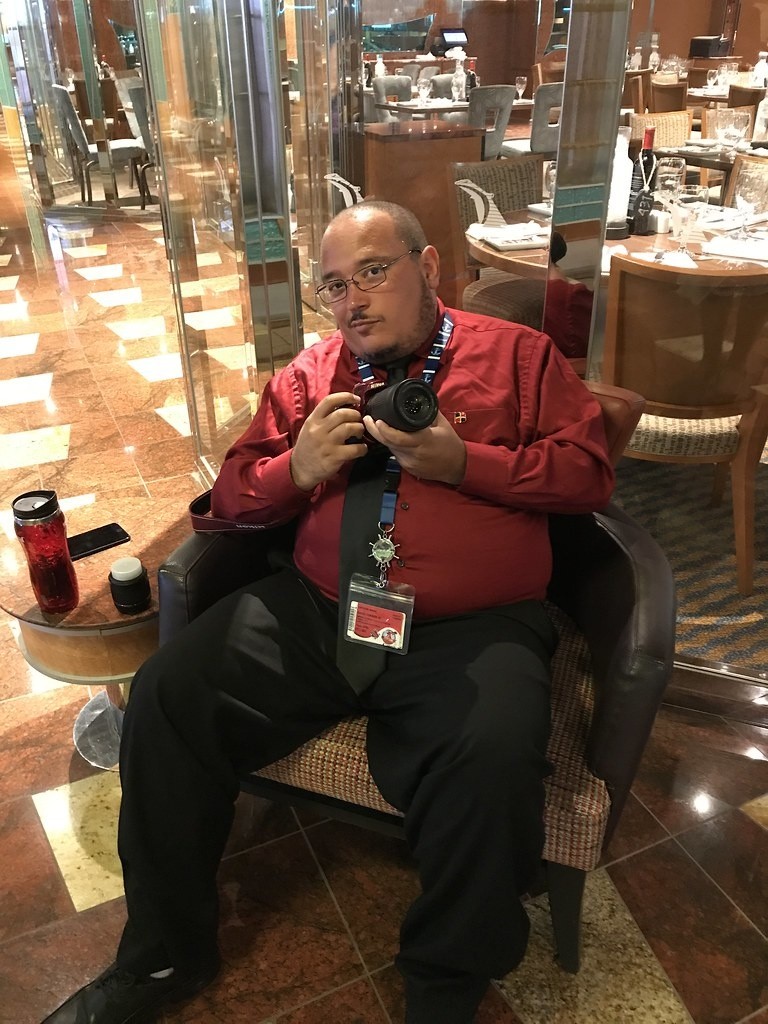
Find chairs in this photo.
[45,34,768,208]
[586,253,768,598]
[158,496,681,974]
[445,149,548,330]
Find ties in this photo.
[335,358,411,696]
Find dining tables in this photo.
[464,198,768,295]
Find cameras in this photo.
[333,377,438,464]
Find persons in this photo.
[42,197,614,1023]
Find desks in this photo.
[2,498,189,775]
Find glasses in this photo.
[315,248,423,304]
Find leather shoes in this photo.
[404,975,486,1024]
[37,952,221,1024]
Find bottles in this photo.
[626,125,658,235]
[101,54,109,79]
[466,60,477,103]
[605,126,634,238]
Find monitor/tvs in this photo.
[440,28,469,48]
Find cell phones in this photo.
[66,523,131,561]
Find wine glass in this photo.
[670,184,709,257]
[516,76,527,104]
[656,158,687,216]
[726,160,768,246]
[108,66,115,78]
[476,76,480,87]
[362,53,467,107]
[63,66,74,90]
[625,41,767,160]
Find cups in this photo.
[10,489,79,614]
